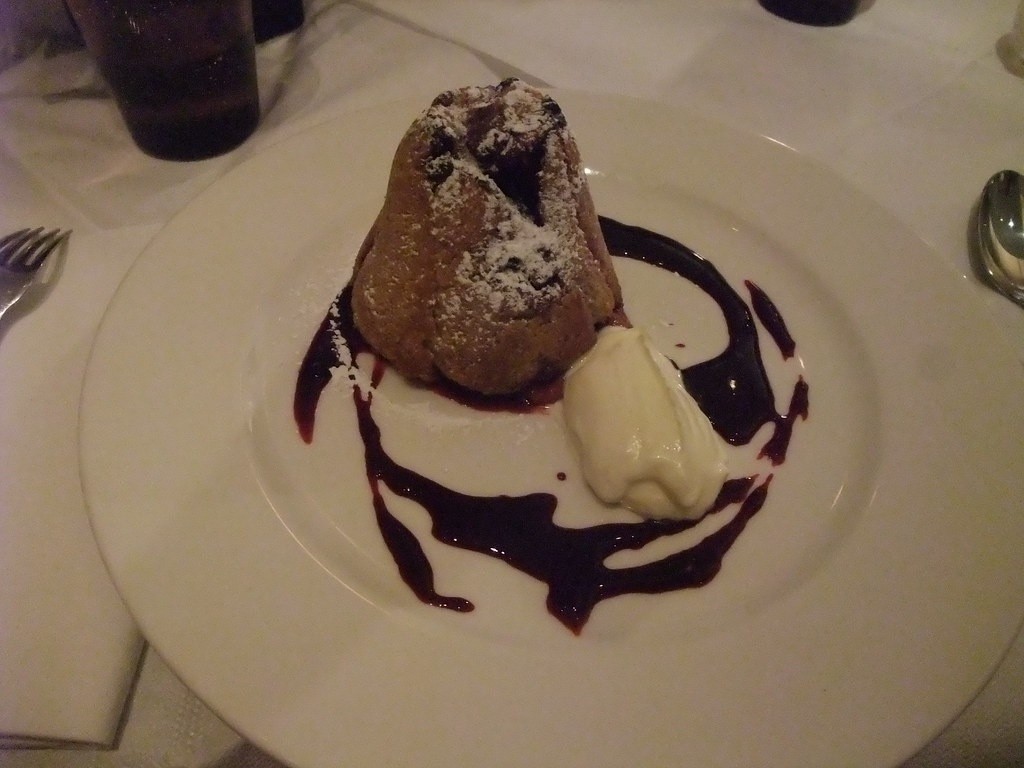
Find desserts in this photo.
[345,73,629,404]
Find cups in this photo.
[65,0,261,163]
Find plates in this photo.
[76,85,1024,768]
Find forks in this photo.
[0,227,75,321]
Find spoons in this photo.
[977,169,1024,306]
